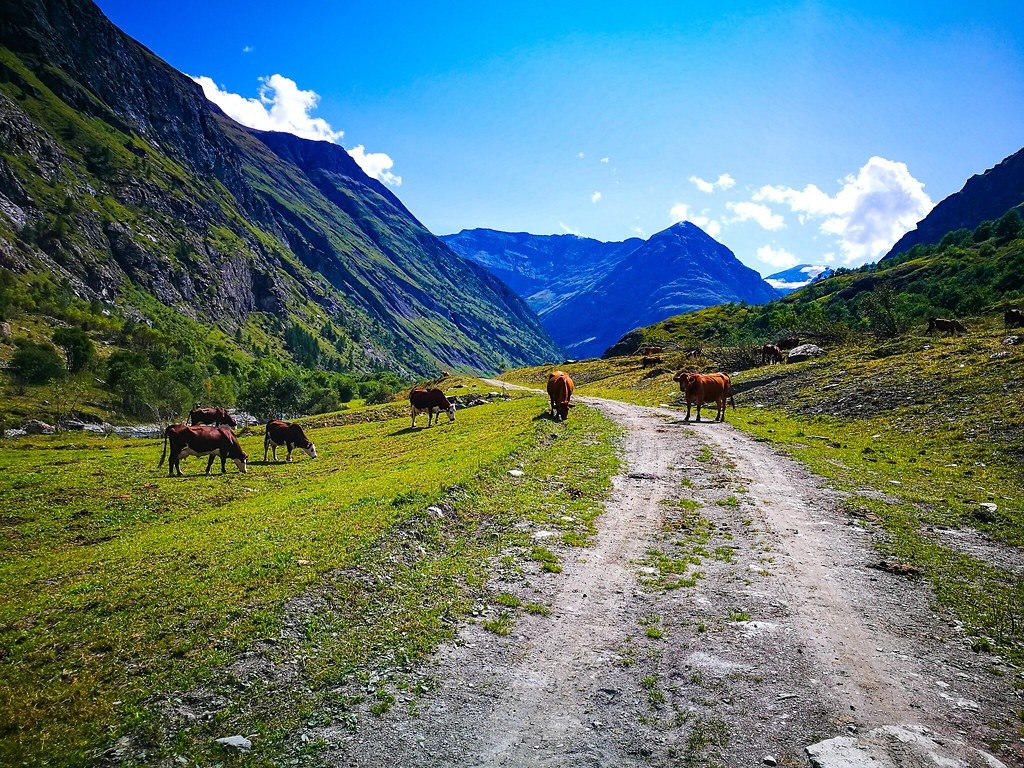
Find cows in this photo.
[673,370,735,423]
[923,316,966,338]
[1004,308,1024,331]
[159,406,317,477]
[409,387,457,428]
[641,356,663,370]
[761,342,785,364]
[547,370,574,419]
